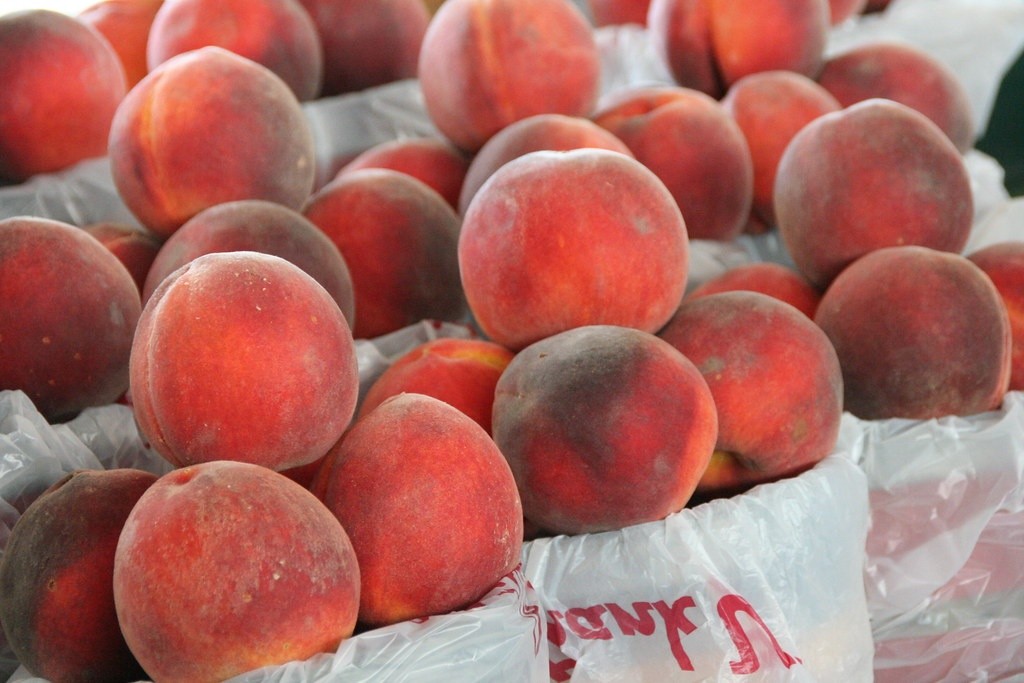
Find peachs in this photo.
[0,0,1024,683]
